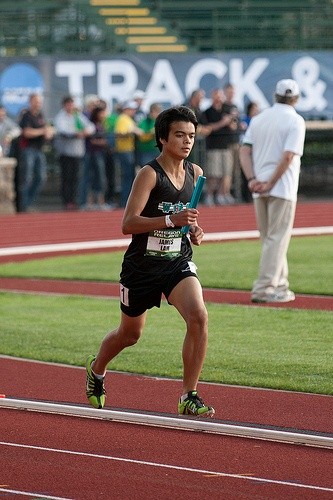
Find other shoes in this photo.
[205,198,214,206]
[225,196,234,205]
[217,197,225,206]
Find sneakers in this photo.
[250,287,289,302]
[85,355,107,409]
[285,288,295,299]
[178,390,215,418]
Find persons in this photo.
[84,106,215,418]
[239,79,306,302]
[0,82,263,215]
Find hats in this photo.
[276,79,300,97]
[122,101,138,109]
[132,89,145,100]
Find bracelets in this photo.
[165,215,174,228]
[247,176,256,182]
